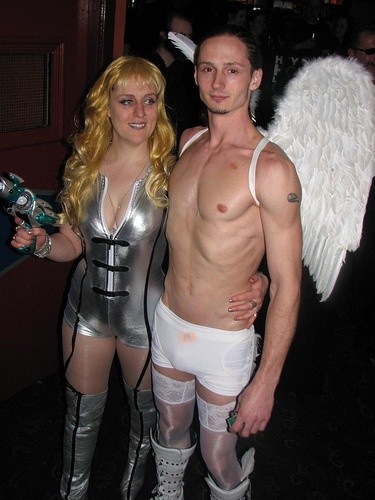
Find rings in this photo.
[253,308,257,313]
[249,300,257,309]
[254,313,257,317]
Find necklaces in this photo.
[102,156,149,219]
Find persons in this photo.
[347,23,375,367]
[11,56,270,500]
[150,27,303,500]
[140,0,356,158]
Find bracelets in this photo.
[34,233,52,259]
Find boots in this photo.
[203,472,251,500]
[119,377,160,499]
[60,384,107,500]
[148,425,197,500]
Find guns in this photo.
[0,171,61,256]
